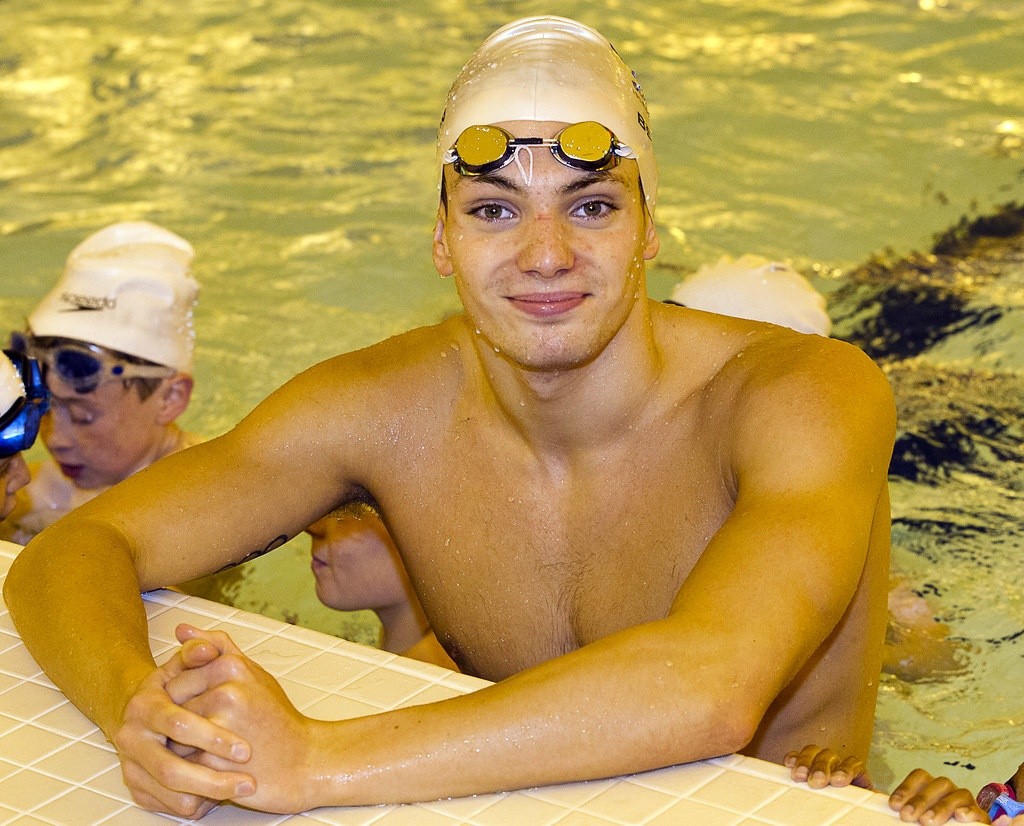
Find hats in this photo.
[26,218,199,379]
[665,250,831,342]
[0,350,29,431]
[433,14,659,226]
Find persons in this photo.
[0,14,896,822]
[0,220,238,601]
[304,254,965,685]
[784,744,1024,826]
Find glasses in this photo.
[1,346,55,458]
[7,330,178,389]
[445,120,633,176]
